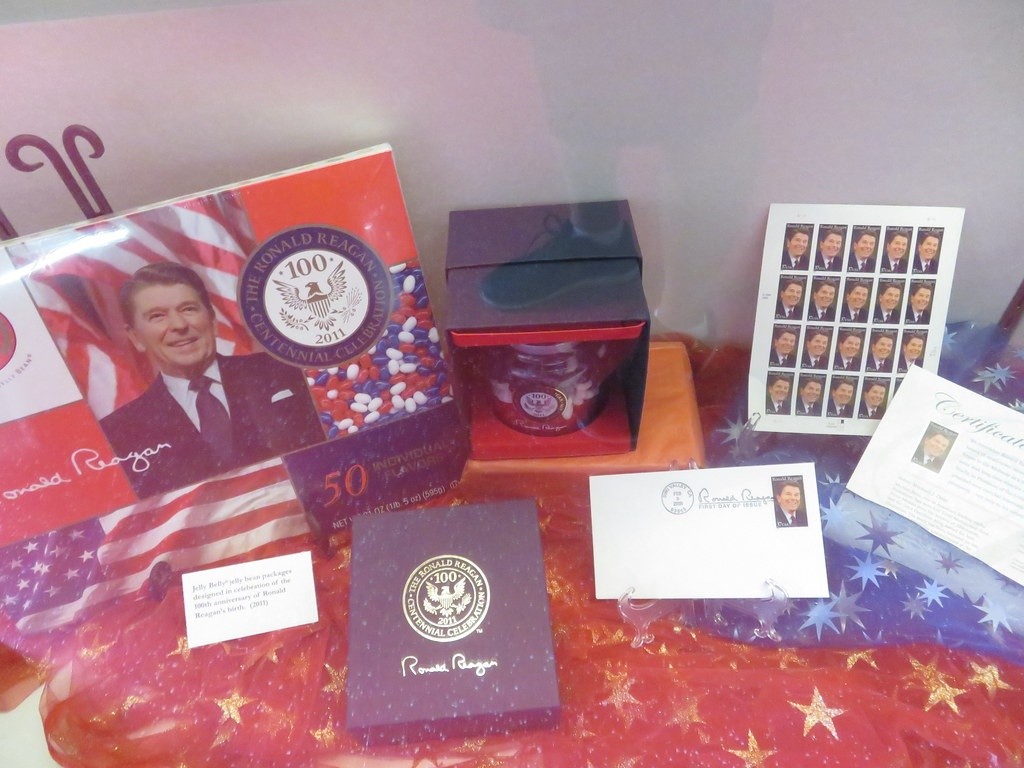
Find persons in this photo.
[775,277,934,325]
[765,372,888,419]
[781,228,942,275]
[99,260,329,502]
[775,480,808,528]
[769,325,926,374]
[911,431,951,473]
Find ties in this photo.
[780,359,885,372]
[777,406,876,418]
[190,369,238,460]
[791,516,797,526]
[787,310,922,324]
[794,259,930,274]
[928,459,931,463]
